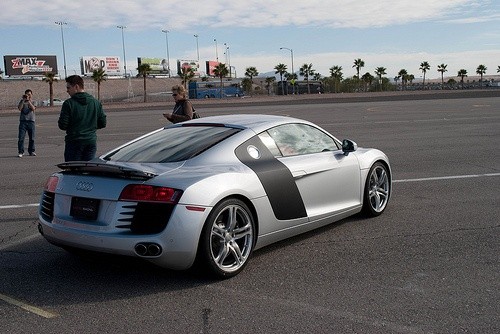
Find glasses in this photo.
[172,93,180,97]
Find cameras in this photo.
[23,95,27,98]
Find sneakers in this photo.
[19,153,23,158]
[31,152,36,155]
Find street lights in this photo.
[280,47,294,94]
[194,34,200,60]
[213,38,218,61]
[116,25,128,76]
[54,21,68,78]
[224,42,230,67]
[162,29,171,77]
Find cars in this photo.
[36,114,392,278]
[42,98,65,107]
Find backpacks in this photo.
[181,100,200,119]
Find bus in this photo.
[188,82,245,99]
[278,80,325,95]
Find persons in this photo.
[58,75,107,162]
[163,84,194,124]
[18,89,38,157]
